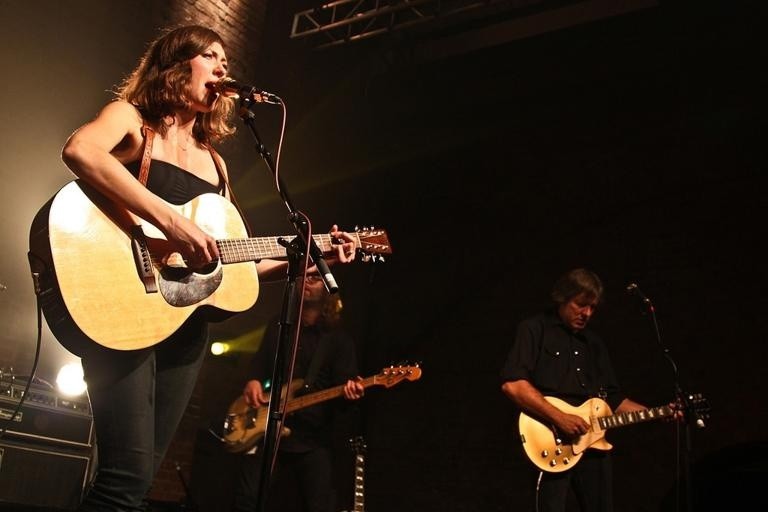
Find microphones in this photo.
[215,77,282,105]
[626,282,655,314]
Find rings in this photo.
[346,251,353,255]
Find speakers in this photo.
[0,437,92,512]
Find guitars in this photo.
[515,392,710,474]
[29,178,393,362]
[222,362,422,456]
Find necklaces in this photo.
[177,126,196,152]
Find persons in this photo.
[59,25,357,512]
[228,255,364,512]
[500,268,684,512]
